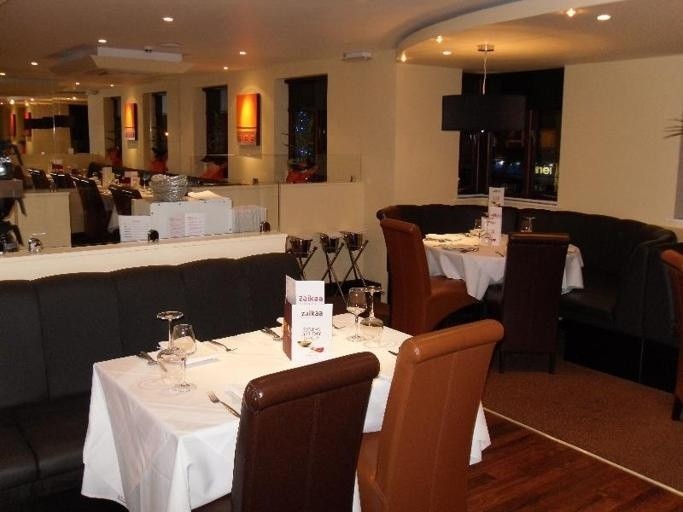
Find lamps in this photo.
[441,46,528,135]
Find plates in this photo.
[425,233,478,254]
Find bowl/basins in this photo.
[148,173,188,202]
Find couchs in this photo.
[1,253,302,512]
[376,204,676,386]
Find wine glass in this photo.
[64,164,148,193]
[357,285,384,348]
[155,309,187,389]
[471,209,535,249]
[347,287,364,341]
[170,318,198,394]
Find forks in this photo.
[205,390,241,421]
[204,337,238,353]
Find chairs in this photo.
[490,231,572,374]
[29,168,142,240]
[227,350,381,512]
[357,318,506,512]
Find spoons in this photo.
[133,348,158,368]
